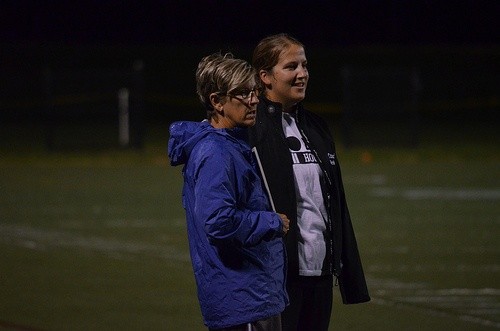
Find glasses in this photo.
[218,85,261,98]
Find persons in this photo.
[166,56,291,331]
[244,33,371,331]
